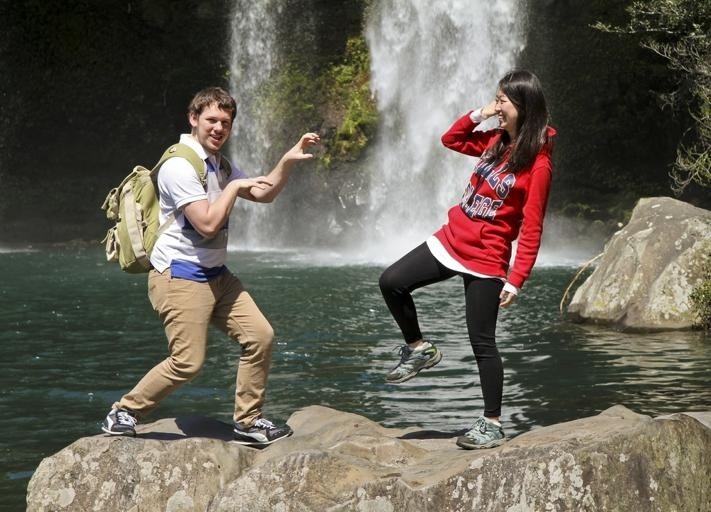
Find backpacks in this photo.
[100,143,207,272]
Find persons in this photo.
[99,86,321,446]
[379,70,558,451]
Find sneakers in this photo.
[229,415,294,445]
[100,404,140,437]
[457,417,507,450]
[385,341,443,385]
